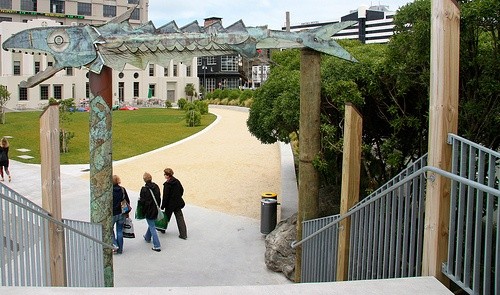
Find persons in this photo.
[0,137,11,183]
[112,174,130,254]
[155,168,187,239]
[140,172,161,252]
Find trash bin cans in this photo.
[261,192,277,200]
[260,198,277,234]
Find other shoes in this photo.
[117,251,122,254]
[179,235,187,239]
[152,248,161,251]
[156,228,165,233]
[113,244,119,252]
[143,234,151,243]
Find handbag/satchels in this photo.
[123,217,135,238]
[157,208,165,221]
[135,200,145,219]
[155,213,168,229]
[178,198,185,208]
[120,186,131,214]
